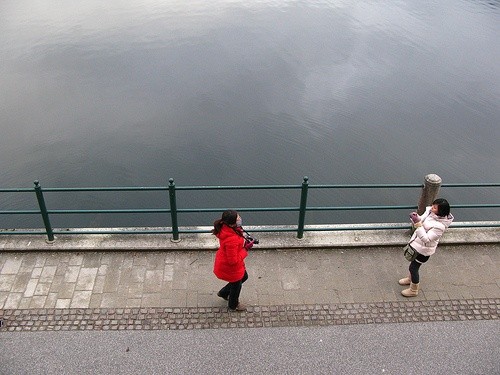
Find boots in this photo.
[401,281,420,296]
[399,272,411,285]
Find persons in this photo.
[211,210,254,312]
[398,198,454,297]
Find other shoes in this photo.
[217,292,229,301]
[228,303,246,311]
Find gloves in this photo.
[245,240,254,250]
[409,212,422,227]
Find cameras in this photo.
[247,236,259,244]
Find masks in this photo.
[236,217,243,227]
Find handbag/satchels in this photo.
[403,244,419,261]
